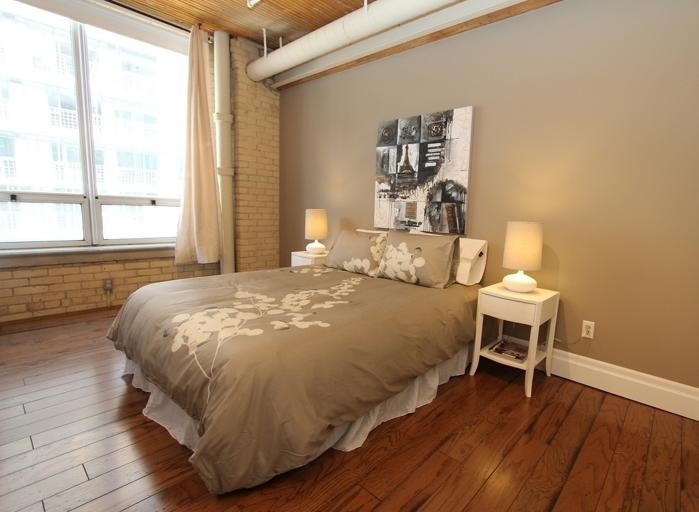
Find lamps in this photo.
[501,221,544,293]
[304,208,327,253]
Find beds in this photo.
[105,229,489,495]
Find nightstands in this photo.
[469,281,560,398]
[290,251,327,268]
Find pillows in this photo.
[321,228,388,279]
[353,227,390,234]
[376,232,461,288]
[406,229,488,286]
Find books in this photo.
[489,338,529,363]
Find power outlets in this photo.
[581,320,596,340]
